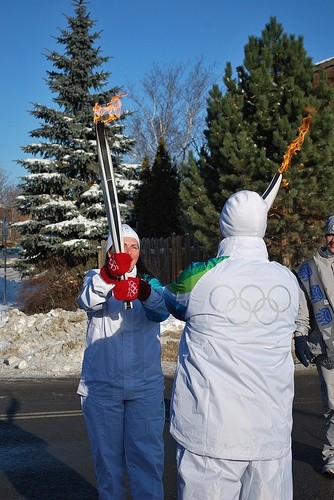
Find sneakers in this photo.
[322,460,334,477]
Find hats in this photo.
[106,224,141,253]
[323,216,334,237]
[219,190,268,238]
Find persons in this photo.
[294,215,334,475]
[78,224,171,500]
[165,190,299,500]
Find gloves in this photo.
[113,277,151,302]
[100,253,134,284]
[294,335,315,367]
